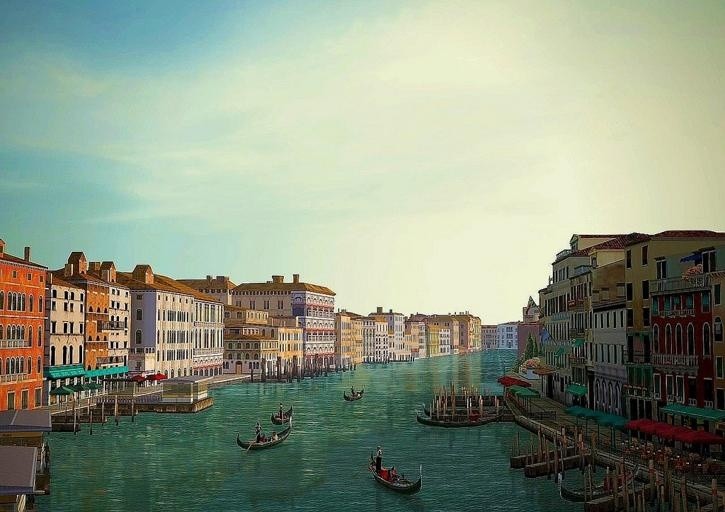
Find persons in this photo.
[392,465,400,482]
[262,433,268,443]
[271,432,278,442]
[375,446,382,474]
[255,422,263,442]
[279,402,284,419]
[387,469,394,482]
[351,385,356,397]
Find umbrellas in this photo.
[565,405,725,474]
[48,373,166,410]
[497,375,541,413]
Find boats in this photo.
[343,385,365,402]
[368,449,424,496]
[236,417,294,450]
[414,407,506,430]
[270,404,294,425]
[554,462,642,503]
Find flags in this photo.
[540,327,551,347]
